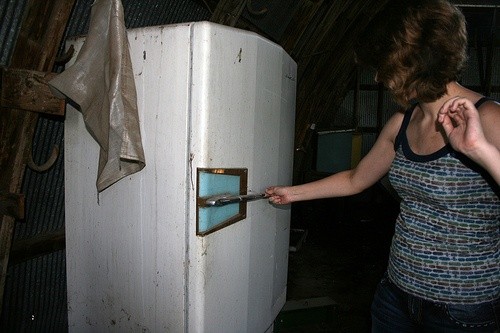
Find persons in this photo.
[263,0,499,333]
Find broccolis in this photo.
[65,20,299,333]
